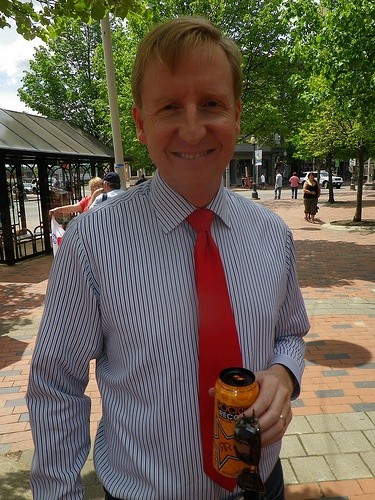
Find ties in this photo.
[186,208,243,492]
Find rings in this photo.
[280,415,286,425]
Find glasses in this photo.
[234,408,263,493]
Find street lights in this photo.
[250,136,258,199]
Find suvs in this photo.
[298,171,343,189]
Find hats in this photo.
[104,172,121,184]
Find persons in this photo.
[303,172,320,223]
[274,170,283,200]
[135,174,148,185]
[91,172,125,210]
[259,173,267,189]
[52,177,59,187]
[24,15,309,500]
[48,177,103,218]
[288,172,299,200]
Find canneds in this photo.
[214,367,259,479]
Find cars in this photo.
[12,178,57,194]
